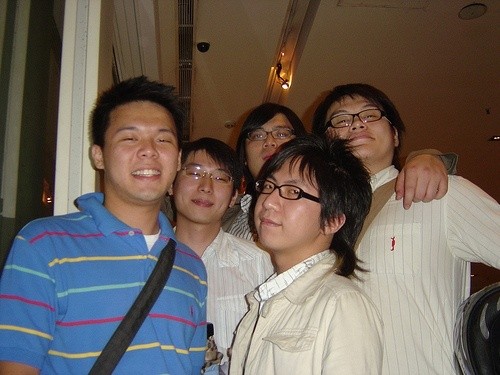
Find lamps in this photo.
[276,50,291,91]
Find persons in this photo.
[171,138,274,375]
[221,102,457,243]
[0,74,208,375]
[312,83,500,375]
[227,127,385,375]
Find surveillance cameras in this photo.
[197,42,210,53]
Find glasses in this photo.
[246,128,296,141]
[179,166,235,185]
[323,109,398,132]
[255,178,322,204]
